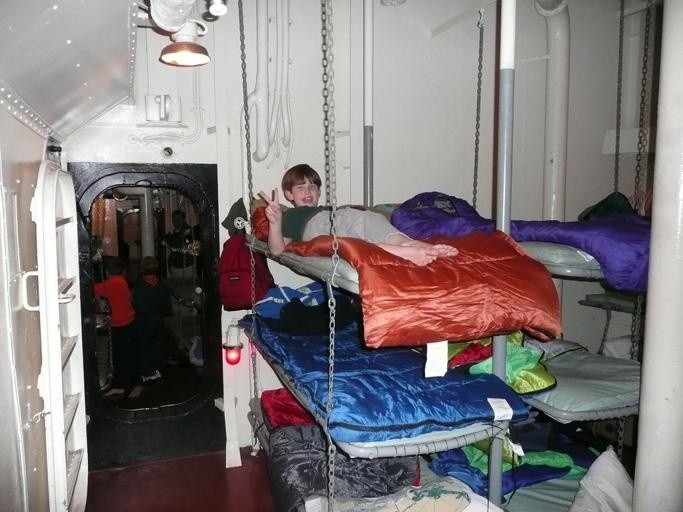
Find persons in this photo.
[166,210,203,281]
[132,256,173,383]
[258,164,459,266]
[94,256,144,397]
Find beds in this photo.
[237,283,534,459]
[454,448,633,512]
[513,221,649,278]
[498,335,644,424]
[247,202,522,296]
[248,387,506,512]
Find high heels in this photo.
[129,386,144,399]
[103,388,125,399]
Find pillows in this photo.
[568,446,634,512]
[260,386,314,428]
[252,206,272,240]
[267,294,356,334]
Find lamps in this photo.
[224,324,244,365]
[160,19,212,68]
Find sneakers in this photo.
[138,370,162,383]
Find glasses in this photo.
[291,184,319,191]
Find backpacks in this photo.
[215,229,275,311]
